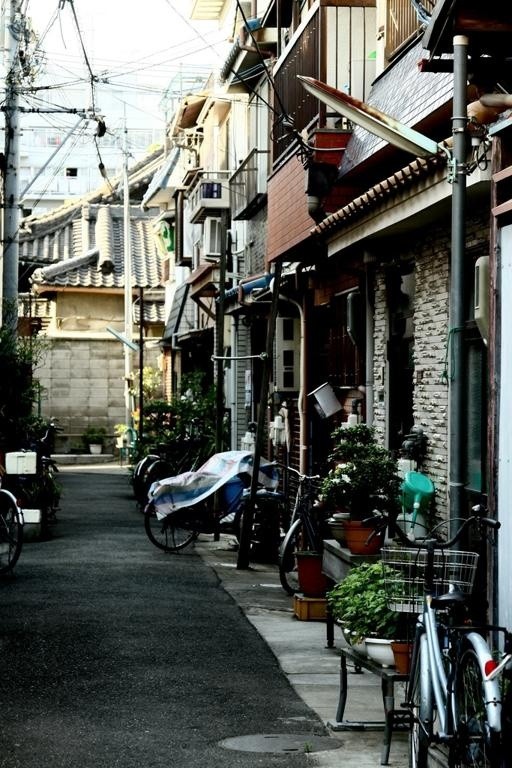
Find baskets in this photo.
[380,548,480,613]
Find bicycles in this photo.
[0,416,65,575]
[131,418,323,596]
[358,504,512,768]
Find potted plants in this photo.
[313,421,406,558]
[324,555,418,670]
[1,294,60,541]
[292,486,336,596]
[134,369,234,515]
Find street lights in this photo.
[296,32,468,576]
[103,282,147,433]
